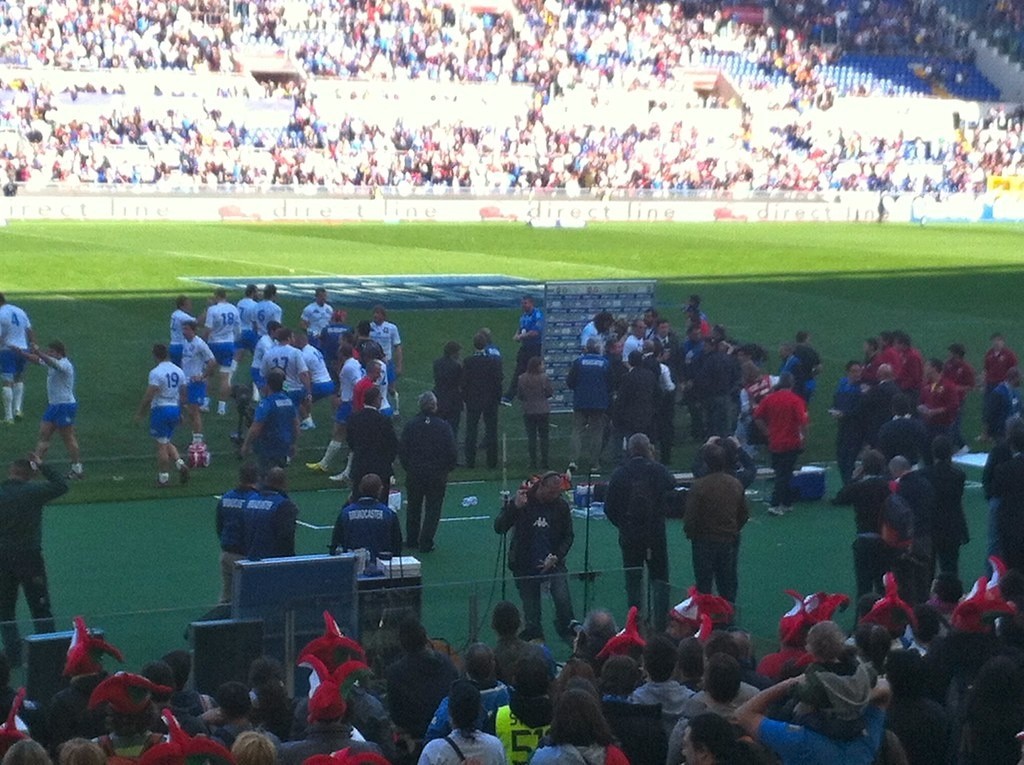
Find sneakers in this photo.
[179,464,192,484]
[306,463,328,473]
[61,469,84,480]
[142,479,170,488]
[329,473,349,481]
[591,468,600,477]
[567,463,578,470]
[299,416,317,430]
[500,396,512,406]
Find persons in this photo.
[0,0,1024,231]
[0,283,1023,765]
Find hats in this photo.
[0,611,391,765]
[596,556,1014,659]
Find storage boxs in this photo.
[790,465,826,500]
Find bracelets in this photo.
[32,345,39,350]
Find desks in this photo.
[675,467,776,502]
[355,570,421,627]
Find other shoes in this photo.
[767,503,794,516]
[217,409,226,415]
[200,407,210,413]
[0,412,23,427]
[230,433,238,440]
